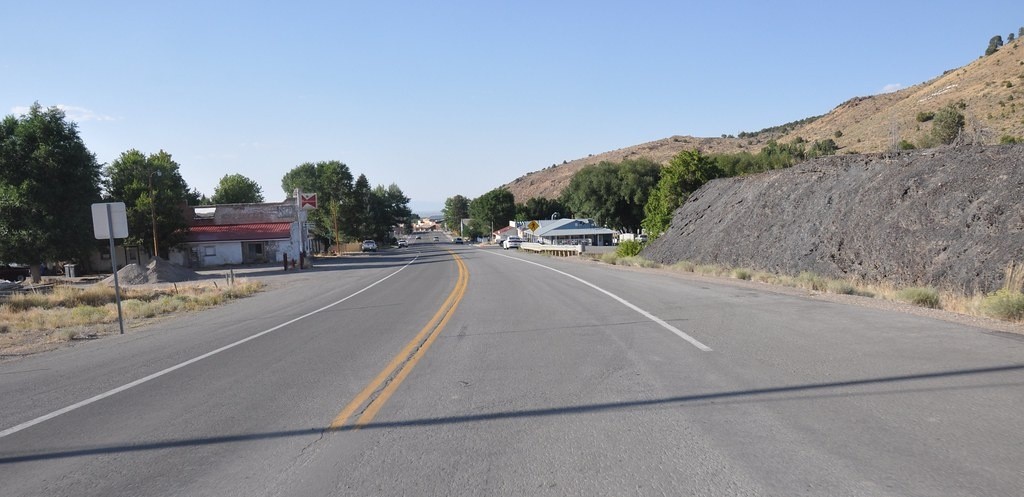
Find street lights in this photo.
[332,195,343,255]
[149,169,163,258]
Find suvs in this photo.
[497,237,522,251]
[456,237,464,244]
[434,236,440,242]
[398,240,408,249]
[362,240,378,252]
[635,235,647,245]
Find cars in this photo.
[416,235,421,239]
[6,264,48,282]
